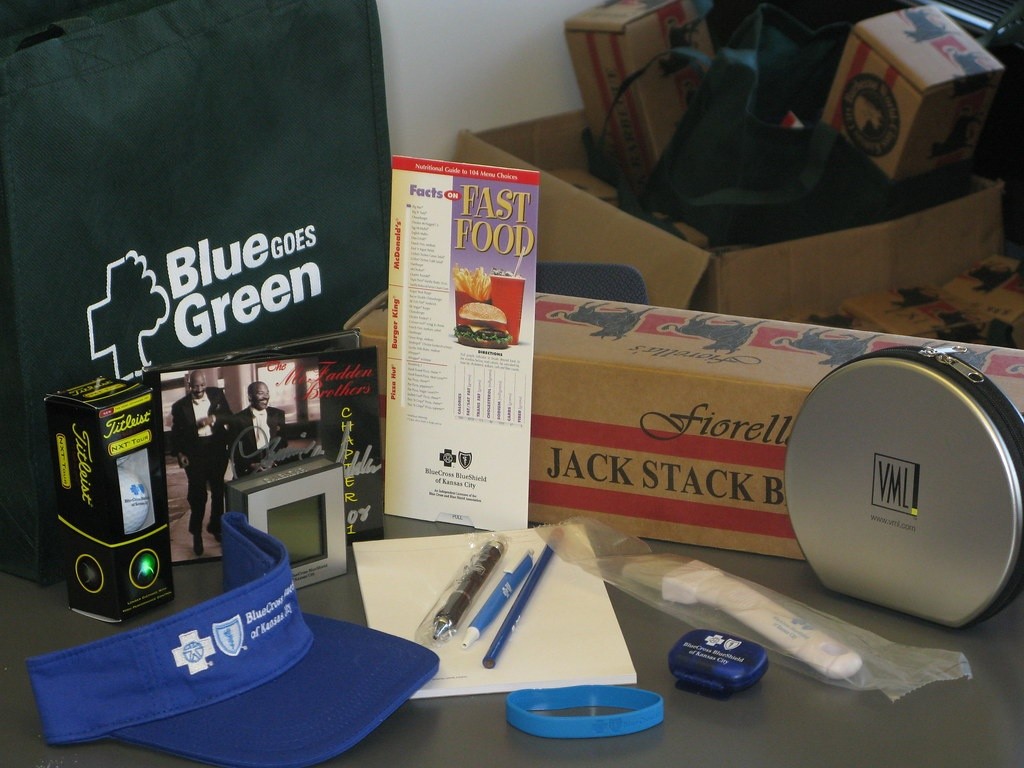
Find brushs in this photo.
[619,555,864,681]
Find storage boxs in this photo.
[453,108,1024,350]
[342,288,1024,562]
[42,378,175,625]
[822,10,1005,183]
[564,0,715,182]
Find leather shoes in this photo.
[192,535,204,556]
[207,524,222,542]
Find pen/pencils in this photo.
[432,539,505,640]
[460,547,535,649]
[482,527,564,669]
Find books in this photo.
[352,525,638,700]
[141,327,385,563]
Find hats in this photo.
[25,511,439,768]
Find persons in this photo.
[171,370,233,556]
[229,381,288,478]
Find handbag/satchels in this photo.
[582,2,978,248]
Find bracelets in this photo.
[499,683,665,737]
